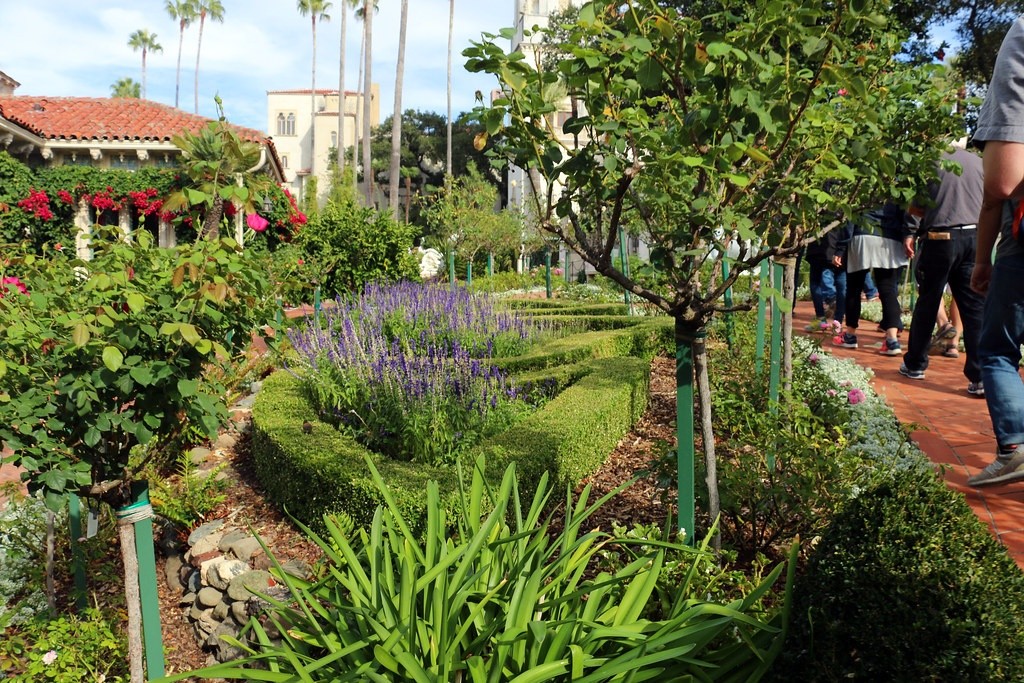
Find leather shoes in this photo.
[968,380,985,394]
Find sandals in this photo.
[927,324,957,356]
[944,343,959,358]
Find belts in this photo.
[951,224,976,229]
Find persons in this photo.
[899,135,993,397]
[783,181,912,357]
[966,15,1024,489]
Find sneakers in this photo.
[967,444,1024,487]
[900,364,925,379]
[832,333,859,348]
[879,339,902,354]
